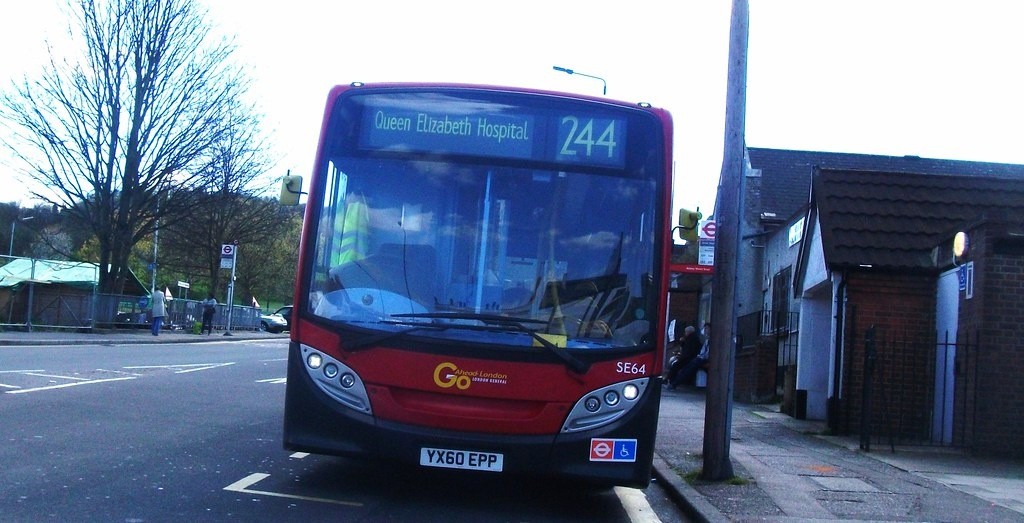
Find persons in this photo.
[663,322,711,389]
[199,291,217,335]
[150,286,168,336]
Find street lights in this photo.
[8,216,33,262]
[553,66,607,97]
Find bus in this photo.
[278,81,702,494]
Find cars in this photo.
[240,306,288,332]
[271,305,293,334]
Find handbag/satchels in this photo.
[699,359,709,373]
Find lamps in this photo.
[952,231,969,258]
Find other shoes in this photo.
[663,385,676,392]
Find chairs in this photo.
[377,243,438,274]
